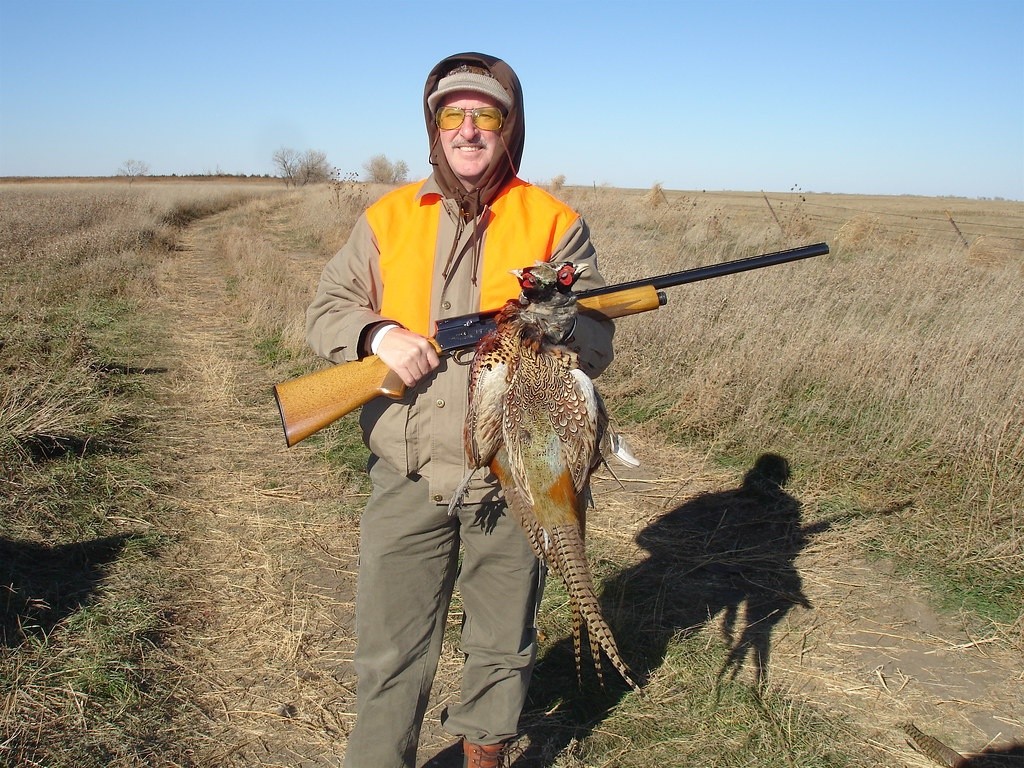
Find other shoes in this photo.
[462,739,523,768]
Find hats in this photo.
[427,65,513,118]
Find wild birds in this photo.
[447,260,657,709]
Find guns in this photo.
[272,242,830,449]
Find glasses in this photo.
[435,105,506,131]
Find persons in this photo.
[304,52,615,768]
